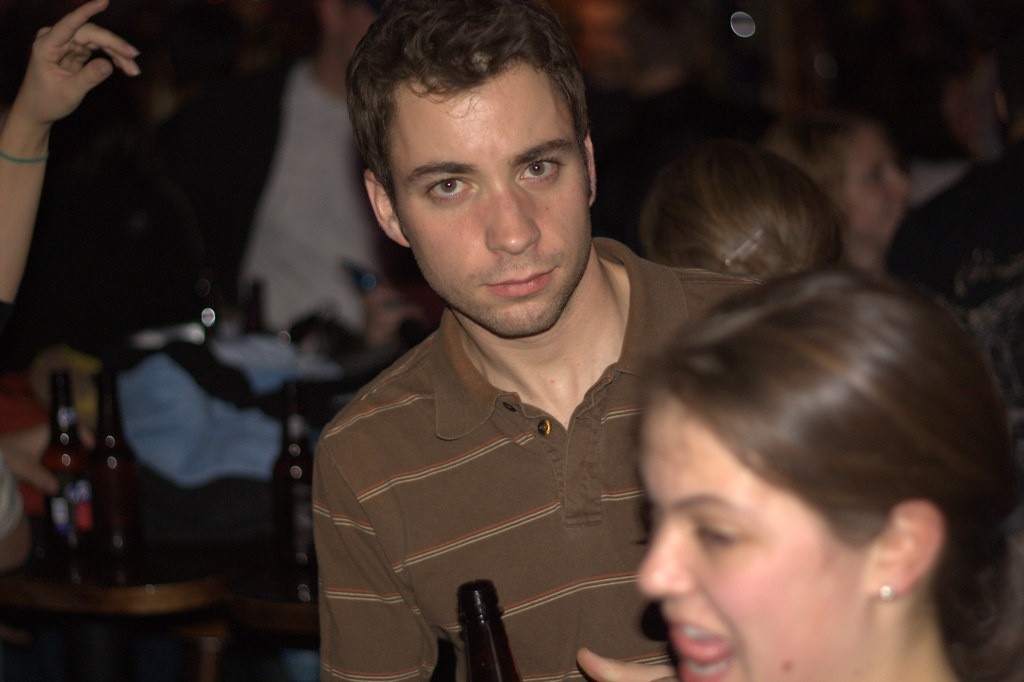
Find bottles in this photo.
[34,368,98,582]
[85,370,148,588]
[270,414,320,604]
[457,578,523,682]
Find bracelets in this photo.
[0,151,48,163]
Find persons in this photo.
[549,0,1024,469]
[0,0,422,682]
[637,269,1024,682]
[311,0,724,681]
